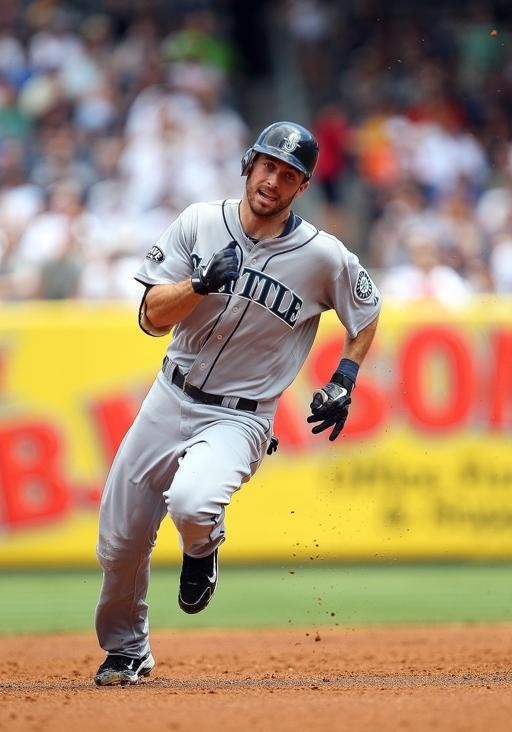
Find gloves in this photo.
[306,372,356,442]
[190,240,241,296]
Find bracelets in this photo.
[334,357,361,384]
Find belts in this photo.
[161,356,258,414]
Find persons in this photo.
[94,119,385,688]
[0,0,511,302]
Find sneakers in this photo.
[178,544,218,614]
[94,648,156,686]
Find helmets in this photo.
[240,121,320,184]
